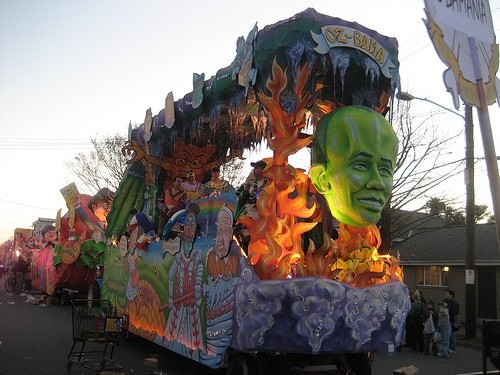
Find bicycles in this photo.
[4,271,15,292]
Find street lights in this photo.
[395,91,478,341]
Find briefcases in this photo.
[392,363,420,375]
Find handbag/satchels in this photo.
[423,312,436,333]
[452,324,460,331]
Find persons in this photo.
[165,177,187,210]
[125,208,154,236]
[32,225,69,252]
[14,256,28,294]
[408,290,460,354]
[201,167,230,198]
[239,161,271,206]
[163,203,200,240]
[67,182,114,243]
[157,199,183,217]
[179,169,202,202]
[309,105,397,228]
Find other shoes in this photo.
[437,353,447,357]
[448,350,456,353]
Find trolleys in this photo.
[65,294,129,375]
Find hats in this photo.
[413,290,420,301]
